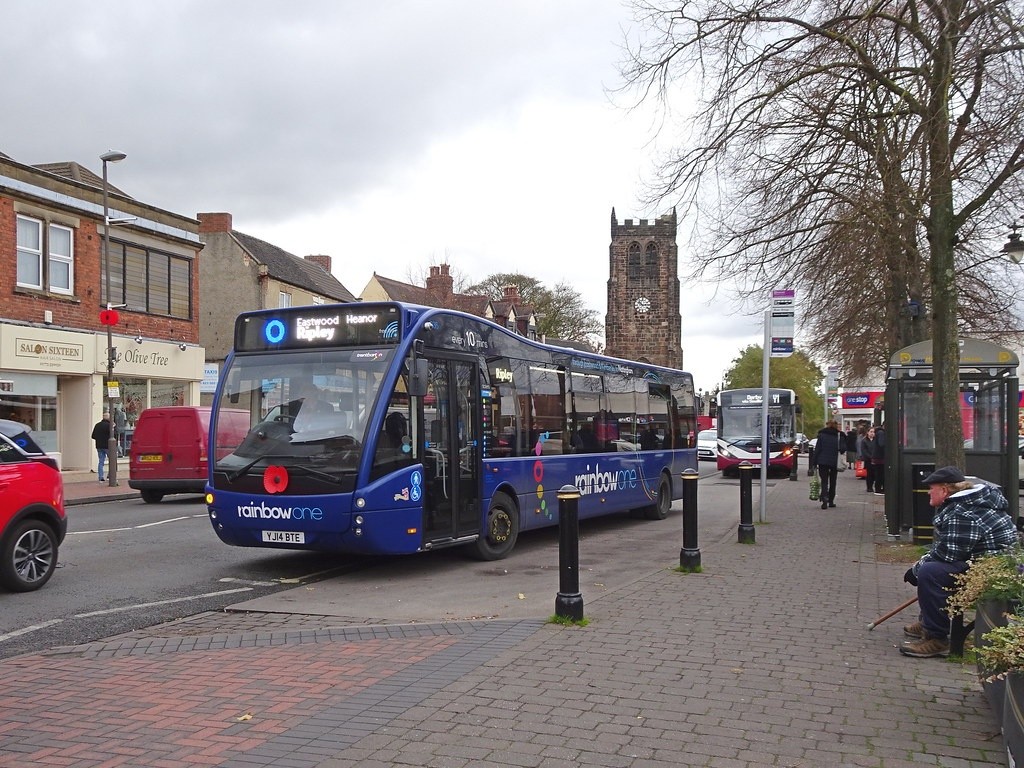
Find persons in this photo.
[290,384,334,431]
[838,424,870,469]
[92,413,110,481]
[1017,410,1024,531]
[511,423,544,454]
[813,420,845,509]
[574,428,671,451]
[861,421,884,495]
[900,466,1018,658]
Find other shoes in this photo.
[868,487,874,492]
[99,478,105,483]
[875,489,885,496]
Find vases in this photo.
[994,668,1023,767]
[963,593,1019,728]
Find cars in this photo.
[807,438,818,452]
[698,426,717,461]
[793,433,810,454]
[0,419,68,592]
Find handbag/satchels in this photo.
[837,452,847,472]
[810,468,819,500]
[856,460,867,477]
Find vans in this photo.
[127,406,251,504]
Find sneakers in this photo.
[900,634,950,658]
[904,621,924,637]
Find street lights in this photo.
[99,150,128,487]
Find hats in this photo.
[922,466,965,483]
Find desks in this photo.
[118,429,133,457]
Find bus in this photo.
[716,387,803,477]
[205,301,700,562]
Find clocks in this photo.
[635,297,650,312]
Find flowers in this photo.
[945,548,1024,609]
[971,618,1023,687]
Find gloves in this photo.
[904,568,918,586]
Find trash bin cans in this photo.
[911,463,935,545]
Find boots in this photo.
[830,499,836,507]
[821,499,827,509]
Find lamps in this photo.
[171,335,187,351]
[123,329,142,345]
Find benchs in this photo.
[941,517,1024,659]
